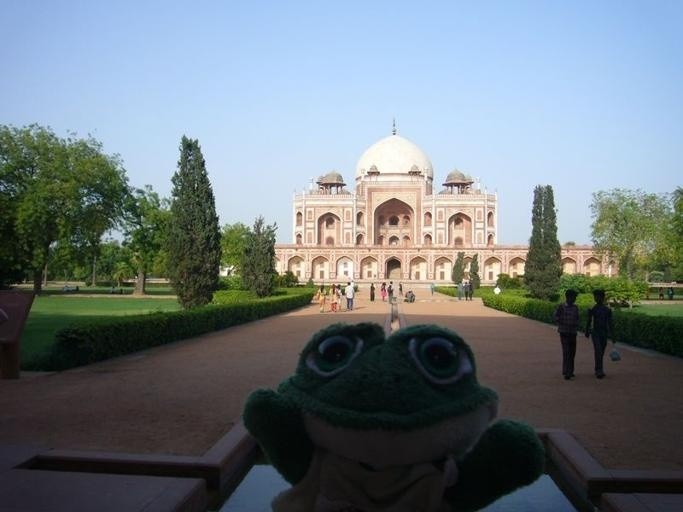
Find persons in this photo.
[493,285,500,295]
[463,282,469,300]
[405,290,413,301]
[658,287,664,300]
[344,282,354,311]
[335,284,341,310]
[468,282,473,300]
[457,281,462,300]
[430,283,434,296]
[585,288,615,379]
[667,287,674,299]
[316,284,326,314]
[369,283,374,301]
[381,281,387,301]
[554,288,579,381]
[398,282,403,295]
[329,283,336,311]
[387,280,393,303]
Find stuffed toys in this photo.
[242,320,547,511]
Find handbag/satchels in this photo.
[608,351,622,361]
